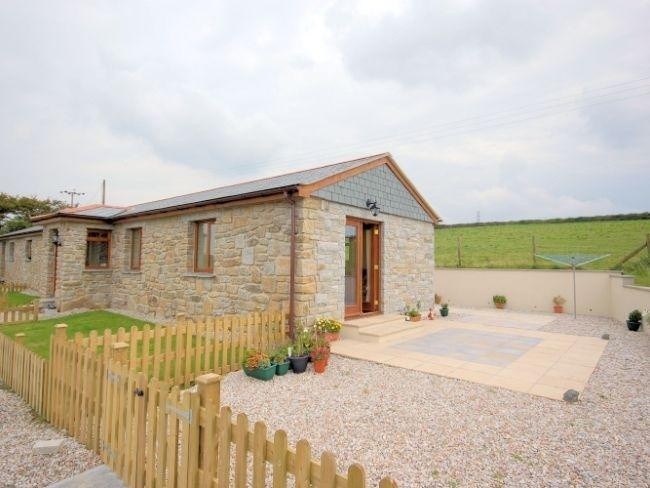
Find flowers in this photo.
[313,316,343,334]
[310,346,330,361]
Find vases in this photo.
[314,359,326,373]
[312,333,341,342]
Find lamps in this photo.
[51,233,61,247]
[366,198,380,217]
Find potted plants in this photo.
[440,303,449,315]
[288,345,308,373]
[626,309,642,331]
[493,294,506,309]
[240,348,276,380]
[552,295,566,313]
[270,352,291,376]
[404,310,422,321]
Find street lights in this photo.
[57,189,86,208]
